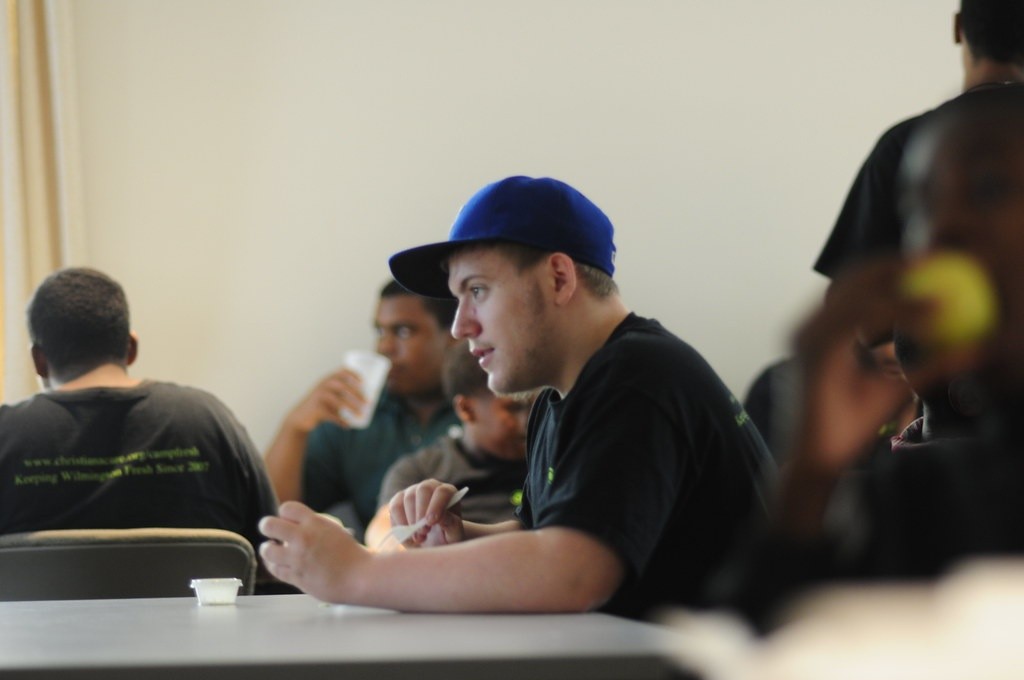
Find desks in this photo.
[0,582,709,680]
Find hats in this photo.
[389,174,616,303]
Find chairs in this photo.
[0,527,259,601]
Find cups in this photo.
[336,350,393,429]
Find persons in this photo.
[814,1,1024,279]
[725,99,1024,593]
[868,343,918,447]
[257,176,782,680]
[378,344,539,524]
[264,279,462,533]
[0,268,284,585]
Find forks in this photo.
[373,485,469,552]
